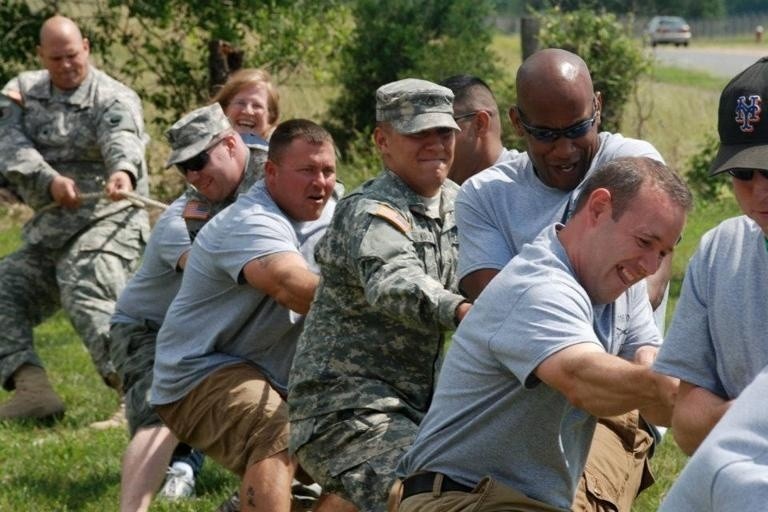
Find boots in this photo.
[0,363,65,426]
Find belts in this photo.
[401,472,472,501]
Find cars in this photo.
[643,16,692,48]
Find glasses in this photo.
[729,168,768,180]
[517,99,597,142]
[175,138,225,174]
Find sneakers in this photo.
[155,467,195,500]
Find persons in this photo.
[290,79,472,512]
[111,68,523,509]
[398,157,691,510]
[0,14,148,430]
[454,50,668,512]
[660,364,768,512]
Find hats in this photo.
[376,78,461,135]
[709,56,768,177]
[164,102,230,169]
